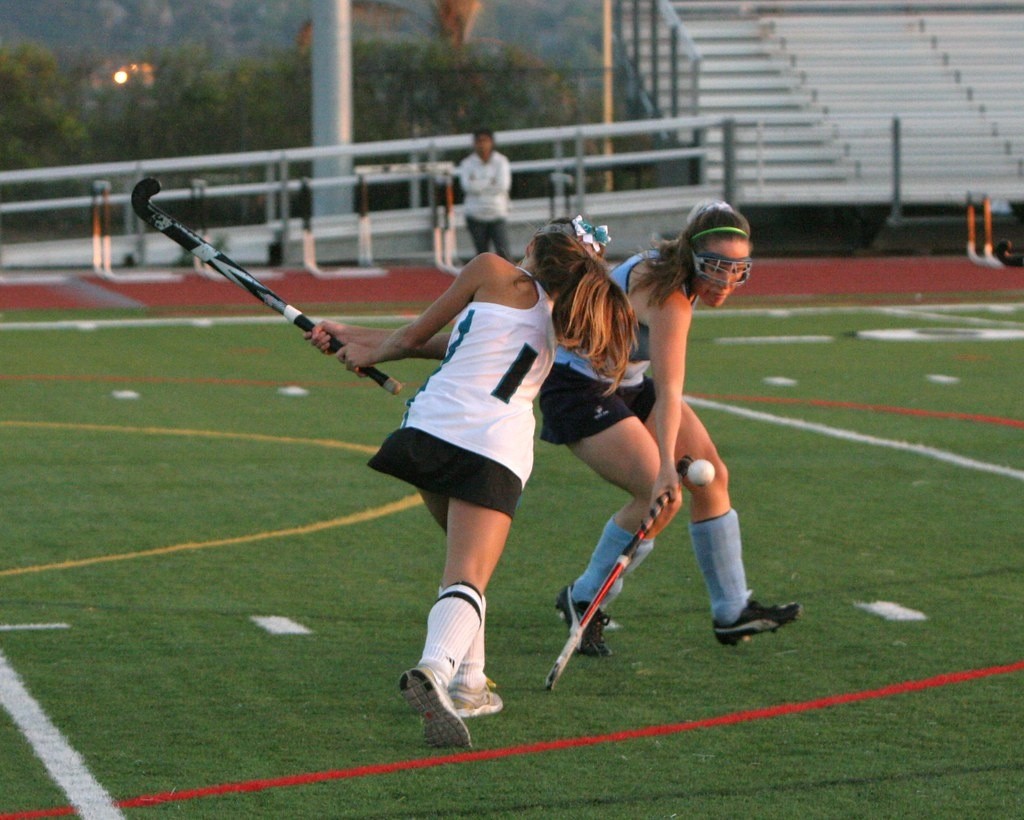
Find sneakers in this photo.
[448,680,504,719]
[713,600,802,647]
[554,584,615,657]
[397,664,474,749]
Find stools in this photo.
[612,0,1023,208]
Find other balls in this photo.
[684,460,715,486]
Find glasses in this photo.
[690,250,753,288]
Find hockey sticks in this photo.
[128,175,404,396]
[542,450,695,693]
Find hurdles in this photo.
[88,179,115,276]
[187,178,217,277]
[963,188,998,261]
[347,155,468,273]
[296,177,323,274]
[548,173,575,226]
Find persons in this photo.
[460,126,513,262]
[300,213,643,748]
[538,198,802,659]
